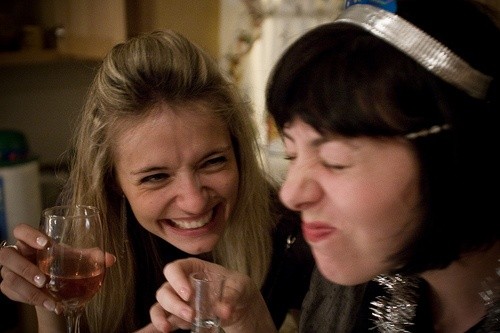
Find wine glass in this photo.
[37,205,105,333]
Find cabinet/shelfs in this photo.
[1,1,129,67]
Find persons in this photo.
[0,28,316,333]
[132,0,500,333]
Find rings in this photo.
[0,240,20,255]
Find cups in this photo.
[189,271,227,333]
